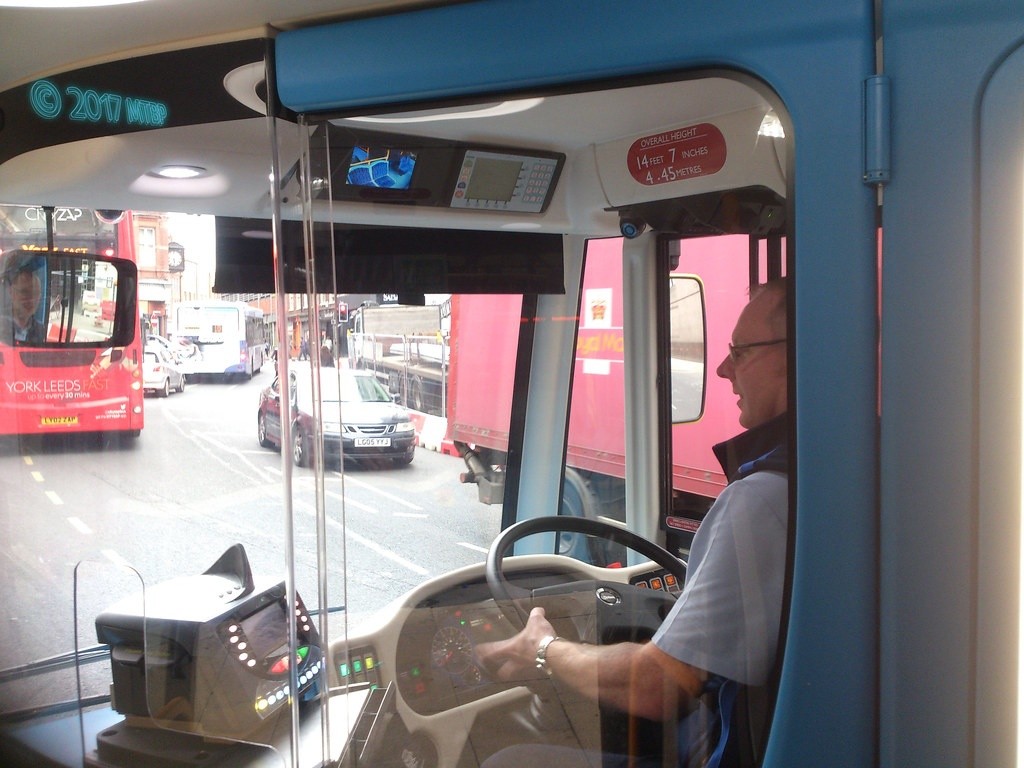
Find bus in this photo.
[1,205,145,436]
[1,0,1018,768]
[440,229,794,558]
[169,299,266,381]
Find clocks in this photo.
[168,242,185,273]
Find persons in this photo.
[0,270,46,342]
[477,281,787,768]
[264,337,332,377]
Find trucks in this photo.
[346,300,450,418]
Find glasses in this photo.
[728,339,783,362]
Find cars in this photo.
[142,344,192,396]
[257,366,416,467]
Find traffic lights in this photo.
[338,303,349,322]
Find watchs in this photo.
[535,636,567,681]
